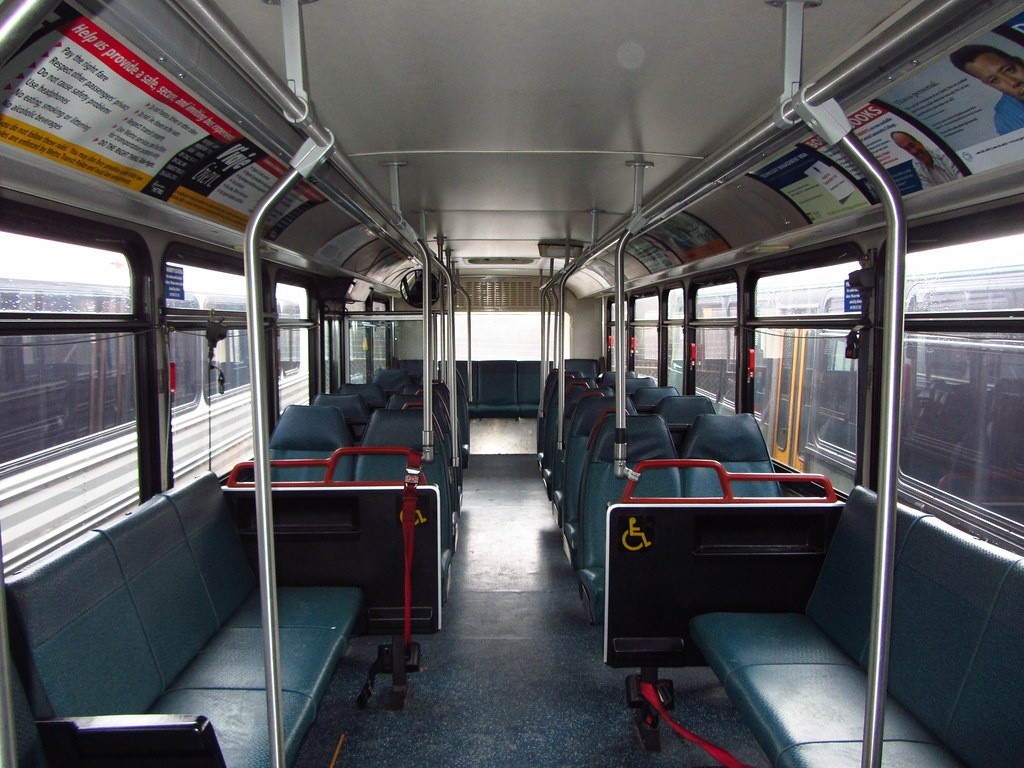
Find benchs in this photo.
[6,470,362,767]
[690,486,1024,768]
[391,357,601,419]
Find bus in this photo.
[630,224,1022,556]
[0,231,386,567]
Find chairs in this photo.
[539,368,784,624]
[909,378,1024,522]
[229,368,467,600]
